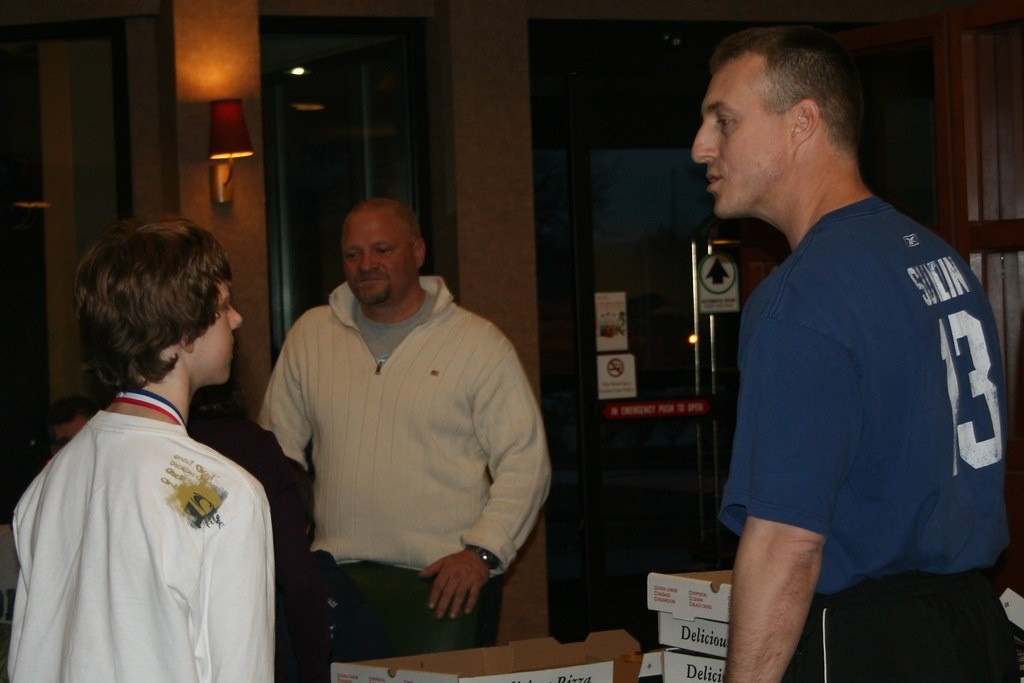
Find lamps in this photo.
[209,98,257,206]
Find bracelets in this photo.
[464,544,500,570]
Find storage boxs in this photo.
[330,569,735,683]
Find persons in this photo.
[258,197,552,657]
[285,455,394,664]
[187,356,332,683]
[690,25,1020,683]
[8,217,276,683]
[46,395,99,457]
